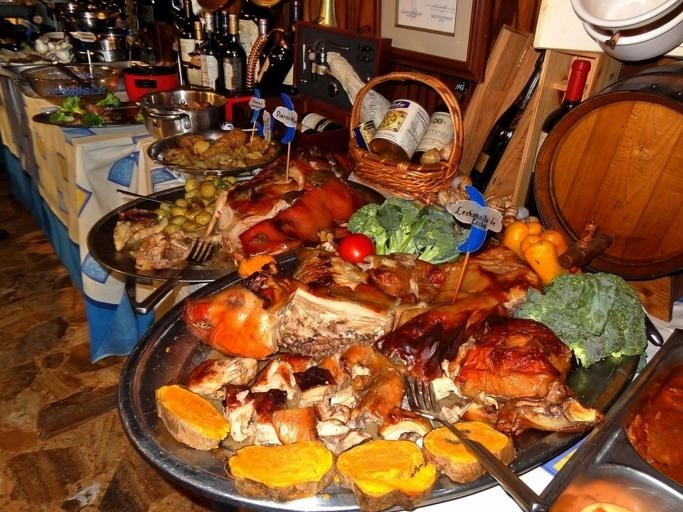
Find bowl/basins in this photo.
[582,11,682,63]
[21,63,119,107]
[570,0,682,30]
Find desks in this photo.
[0,67,186,364]
[142,135,681,511]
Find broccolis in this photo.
[82,110,102,125]
[57,95,83,114]
[96,92,117,106]
[515,272,649,367]
[346,196,461,266]
[49,110,75,123]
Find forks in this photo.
[133,236,215,316]
[403,376,545,512]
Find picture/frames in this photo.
[369,1,491,85]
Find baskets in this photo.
[346,71,463,206]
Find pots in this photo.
[133,85,227,140]
[121,67,179,103]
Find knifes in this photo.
[53,62,95,92]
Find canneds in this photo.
[351,121,377,153]
[369,99,430,160]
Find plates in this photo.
[145,128,284,177]
[31,101,145,128]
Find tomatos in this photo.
[341,233,376,267]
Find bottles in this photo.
[315,0,337,28]
[412,79,467,164]
[296,111,339,135]
[289,1,304,26]
[175,1,271,100]
[524,55,592,219]
[256,24,295,89]
[468,52,545,195]
